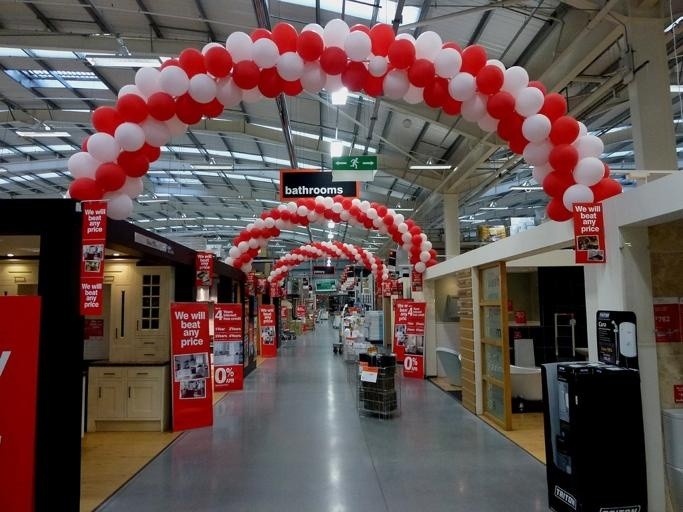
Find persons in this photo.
[84,246,99,271]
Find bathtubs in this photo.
[491,364,543,402]
[435,348,462,387]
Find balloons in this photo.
[223,196,437,275]
[265,240,389,283]
[65,16,624,222]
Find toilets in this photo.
[661,407,683,504]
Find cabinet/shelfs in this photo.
[78,266,174,433]
